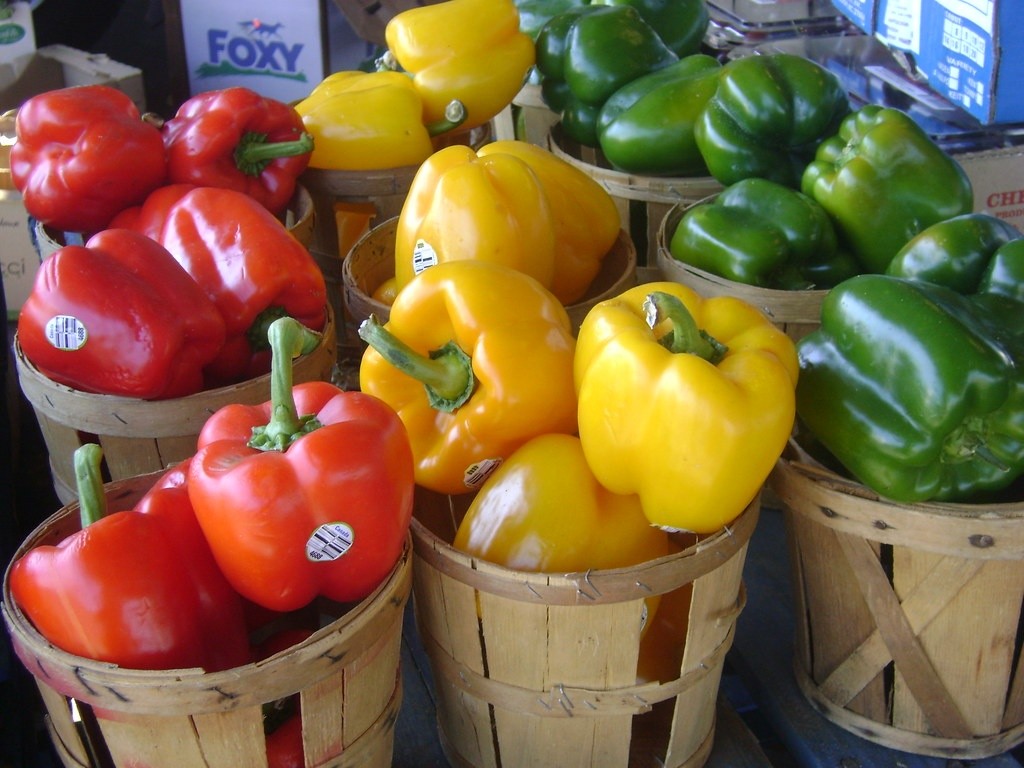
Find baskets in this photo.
[1,81,1024,768]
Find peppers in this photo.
[1,0,1024,768]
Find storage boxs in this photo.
[0,1,1023,334]
